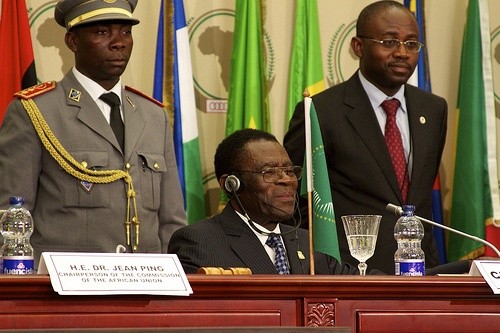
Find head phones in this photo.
[224,174,303,236]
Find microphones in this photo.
[384,203,500,257]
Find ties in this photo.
[100,93,125,154]
[379,98,408,203]
[267,234,292,274]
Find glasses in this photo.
[242,165,309,183]
[361,36,423,53]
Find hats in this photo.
[54,0,140,31]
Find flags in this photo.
[286,0,325,128]
[0,0,38,130]
[226,0,265,137]
[404,0,448,266]
[152,0,204,226]
[299,98,341,264]
[448,0,500,265]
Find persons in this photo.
[283,0,449,272]
[0,0,188,266]
[168,129,386,275]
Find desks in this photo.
[0,274,500,333]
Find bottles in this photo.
[0,195,34,274]
[393,204,425,276]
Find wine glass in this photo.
[340,214,382,276]
